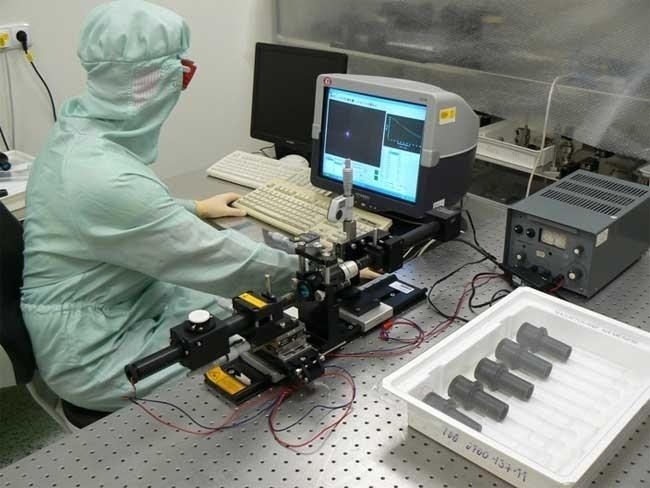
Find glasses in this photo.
[180,57,198,91]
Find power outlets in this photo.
[1,22,31,50]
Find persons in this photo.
[20,0,301,412]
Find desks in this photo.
[0,145,648,488]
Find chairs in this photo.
[0,200,117,437]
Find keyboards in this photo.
[231,178,393,256]
[207,150,334,198]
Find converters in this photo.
[504,266,551,294]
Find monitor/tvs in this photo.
[309,72,480,223]
[248,40,347,160]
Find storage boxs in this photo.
[380,285,649,488]
